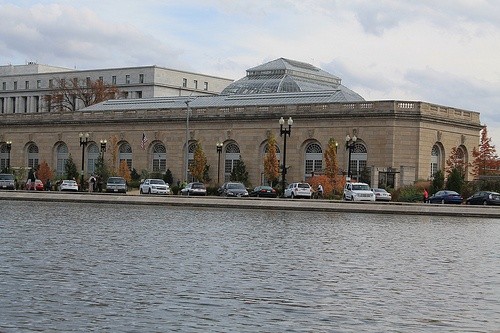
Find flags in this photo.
[140,133,148,149]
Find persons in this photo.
[46,178,63,190]
[31,175,36,189]
[310,184,316,198]
[318,183,323,195]
[73,175,86,191]
[88,174,103,193]
[423,189,428,203]
[177,179,187,188]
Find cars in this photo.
[106,177,127,193]
[249,186,277,199]
[57,180,78,192]
[465,190,500,206]
[139,179,171,195]
[181,182,208,196]
[283,183,314,199]
[26,179,44,191]
[222,183,249,197]
[428,190,464,204]
[371,188,392,202]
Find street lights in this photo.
[78,132,90,191]
[345,134,357,183]
[6,141,12,169]
[100,140,107,167]
[278,116,293,198]
[216,142,224,184]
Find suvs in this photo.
[0,173,16,190]
[342,182,376,202]
